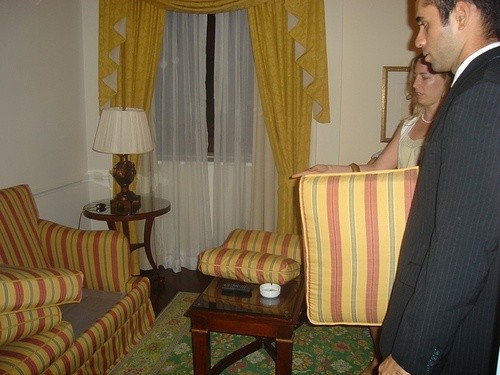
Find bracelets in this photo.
[349,164,360,172]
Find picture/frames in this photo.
[381,64,415,144]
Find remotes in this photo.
[221,282,252,295]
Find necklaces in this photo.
[422,114,433,123]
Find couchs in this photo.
[0,184,156,375]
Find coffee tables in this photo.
[184,272,307,374]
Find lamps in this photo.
[91,107,153,213]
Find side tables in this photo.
[82,196,171,292]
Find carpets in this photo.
[105,291,377,374]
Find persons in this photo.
[378,0,500,375]
[291,54,453,375]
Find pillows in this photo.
[0,321,75,375]
[195,229,306,281]
